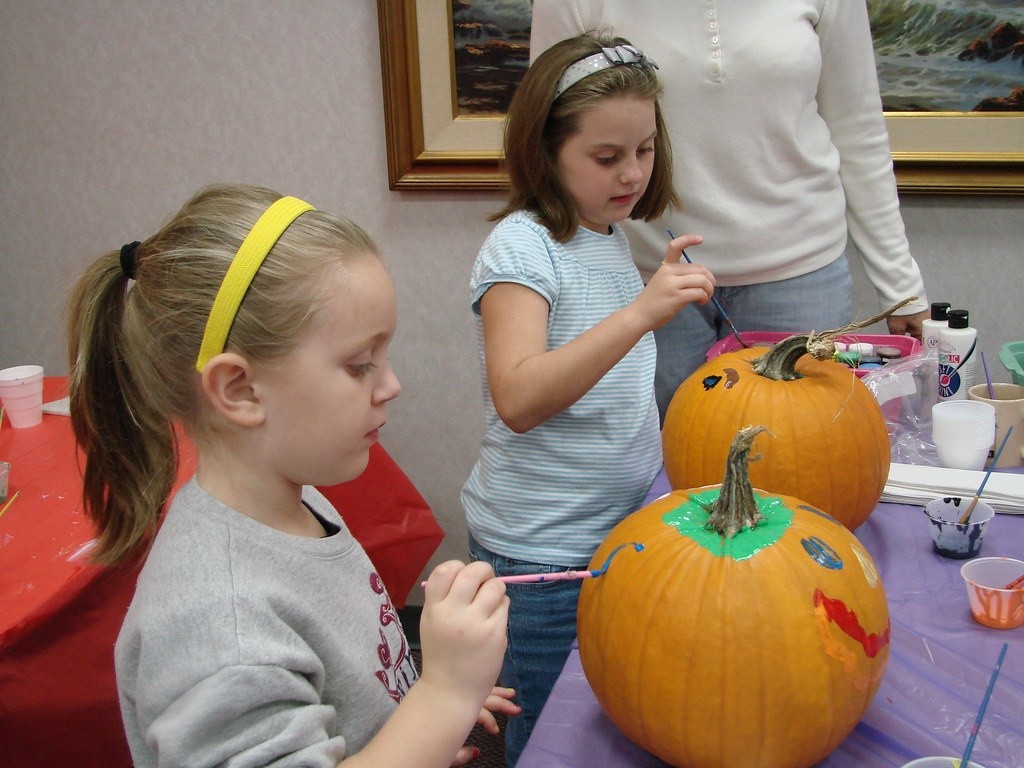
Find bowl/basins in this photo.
[932,399,995,470]
[0,461,11,506]
[924,496,995,559]
[960,556,1024,629]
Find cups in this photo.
[0,365,44,429]
[968,382,1024,468]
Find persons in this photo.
[458,27,716,767]
[528,0,933,429]
[68,183,522,767]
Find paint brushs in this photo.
[665,228,751,349]
[959,426,1013,524]
[0,490,20,516]
[958,641,1008,768]
[419,559,611,590]
[980,350,995,399]
[1005,574,1024,589]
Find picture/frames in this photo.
[364,0,1023,198]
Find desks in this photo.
[0,366,446,768]
[508,459,1024,768]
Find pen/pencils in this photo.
[0,406,5,428]
